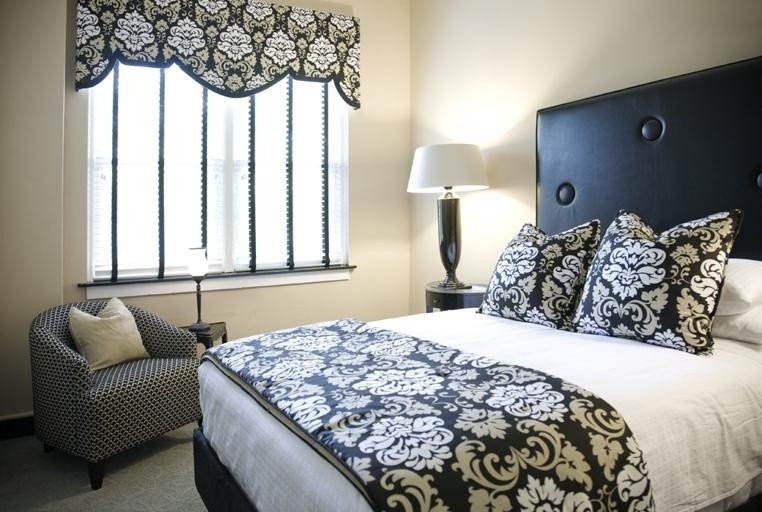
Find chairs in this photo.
[28,298,201,489]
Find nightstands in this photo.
[426,285,488,312]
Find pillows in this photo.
[63,297,151,373]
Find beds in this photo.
[188,52,761,511]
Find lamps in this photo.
[405,142,491,291]
[186,248,211,332]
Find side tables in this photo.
[177,321,227,349]
